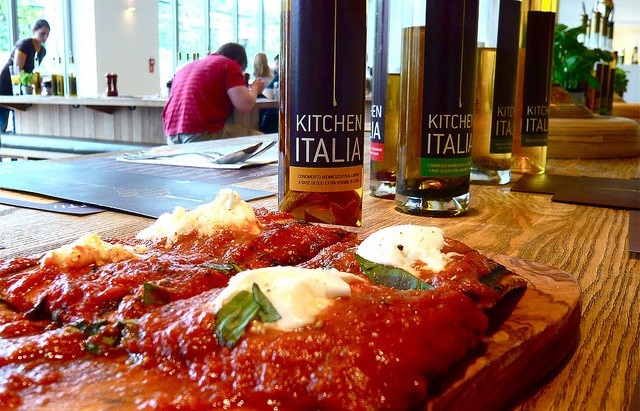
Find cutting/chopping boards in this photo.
[1,221,582,411]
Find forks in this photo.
[137,142,278,162]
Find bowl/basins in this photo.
[262,89,278,99]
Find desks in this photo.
[0,94,278,144]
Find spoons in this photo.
[121,142,263,164]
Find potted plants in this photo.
[549,22,613,105]
[19,73,33,95]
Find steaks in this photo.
[1,187,640,411]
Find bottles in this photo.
[50,54,57,95]
[368,1,405,201]
[395,0,480,218]
[469,1,521,188]
[580,7,618,117]
[57,55,65,96]
[510,1,558,176]
[277,0,366,228]
[33,54,44,95]
[68,53,77,97]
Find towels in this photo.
[116,141,280,168]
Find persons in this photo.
[259,54,279,134]
[162,42,265,146]
[253,53,275,86]
[0,19,51,161]
